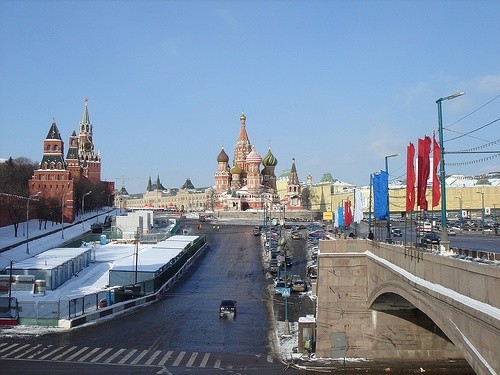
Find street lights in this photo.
[61,190,73,239]
[81,191,92,231]
[435,90,467,249]
[385,153,401,243]
[26,192,42,255]
[251,193,290,336]
[453,196,462,230]
[475,191,486,231]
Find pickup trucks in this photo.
[219,299,238,318]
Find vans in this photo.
[416,222,432,232]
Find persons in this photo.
[368,230,374,240]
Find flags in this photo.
[432,135,442,208]
[405,135,431,215]
[371,169,394,220]
[333,190,365,229]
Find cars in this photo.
[389,227,403,237]
[272,274,307,295]
[305,224,327,278]
[420,233,441,245]
[253,225,263,236]
[446,228,457,237]
[389,216,405,223]
[266,225,282,273]
[362,217,382,228]
[285,224,306,240]
[283,256,292,267]
